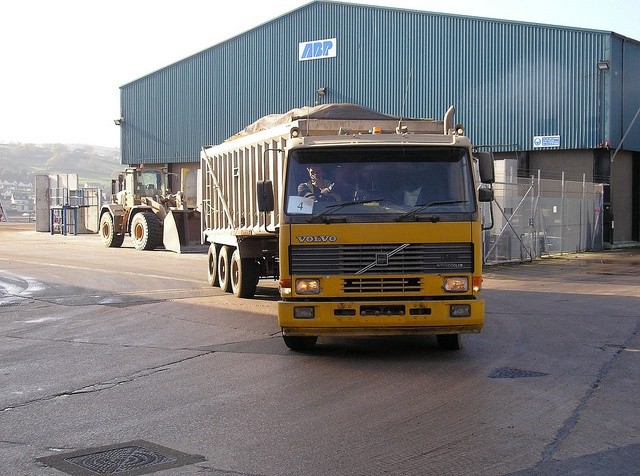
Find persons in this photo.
[298,164,342,203]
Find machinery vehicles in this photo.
[98,164,209,254]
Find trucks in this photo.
[199,103,496,352]
